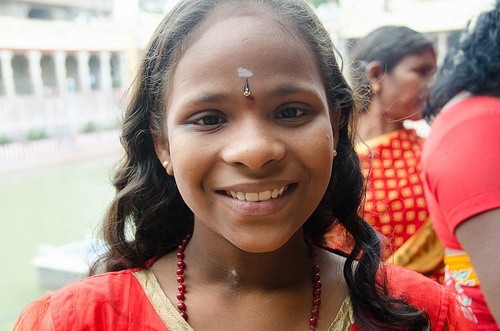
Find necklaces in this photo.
[176,234,323,331]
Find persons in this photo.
[14,0,474,331]
[421,0,500,331]
[321,26,446,288]
